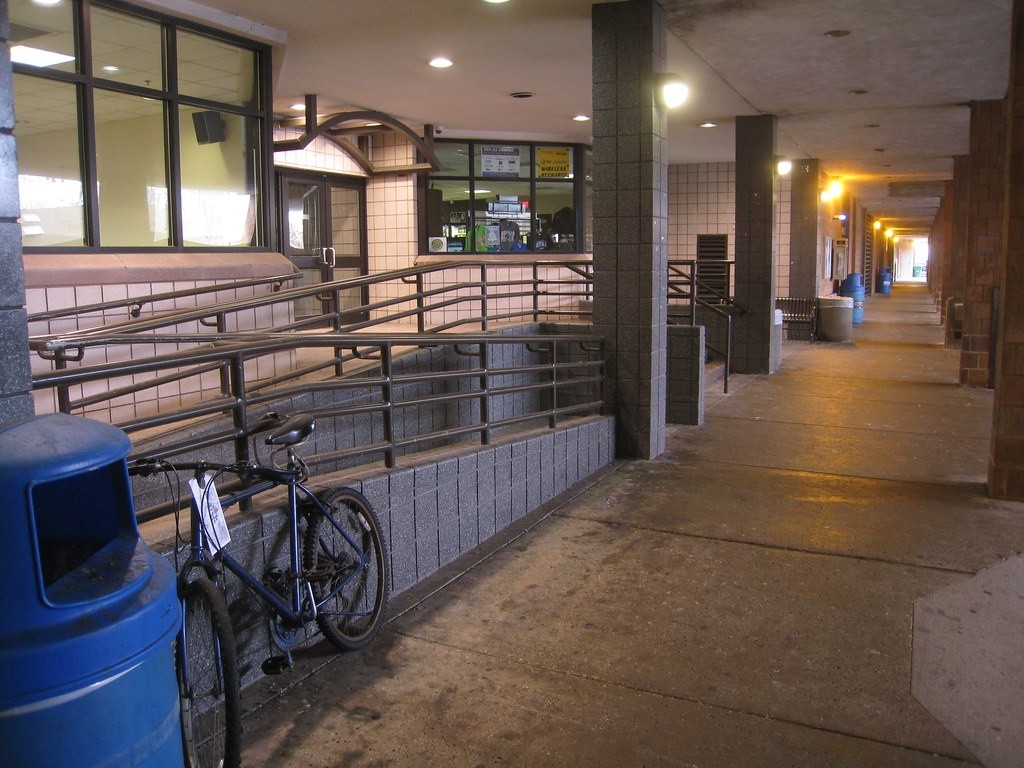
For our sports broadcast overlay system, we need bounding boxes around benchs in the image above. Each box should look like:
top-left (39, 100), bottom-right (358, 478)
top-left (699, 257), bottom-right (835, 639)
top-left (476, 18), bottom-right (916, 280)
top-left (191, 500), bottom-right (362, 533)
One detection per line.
top-left (773, 297), bottom-right (819, 343)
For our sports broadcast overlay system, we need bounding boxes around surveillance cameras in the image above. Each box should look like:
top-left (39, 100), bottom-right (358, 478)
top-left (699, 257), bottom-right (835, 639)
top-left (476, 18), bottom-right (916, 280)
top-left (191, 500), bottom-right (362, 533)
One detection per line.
top-left (435, 126), bottom-right (443, 134)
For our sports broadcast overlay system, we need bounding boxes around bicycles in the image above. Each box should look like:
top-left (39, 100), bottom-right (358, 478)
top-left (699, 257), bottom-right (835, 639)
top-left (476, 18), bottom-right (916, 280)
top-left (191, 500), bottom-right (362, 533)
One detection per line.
top-left (123, 413), bottom-right (391, 768)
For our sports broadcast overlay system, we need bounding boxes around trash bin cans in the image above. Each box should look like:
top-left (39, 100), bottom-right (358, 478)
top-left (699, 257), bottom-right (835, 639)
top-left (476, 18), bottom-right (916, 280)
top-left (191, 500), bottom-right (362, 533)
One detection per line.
top-left (879, 267), bottom-right (892, 294)
top-left (913, 265), bottom-right (922, 277)
top-left (838, 273), bottom-right (865, 324)
top-left (0, 413), bottom-right (185, 768)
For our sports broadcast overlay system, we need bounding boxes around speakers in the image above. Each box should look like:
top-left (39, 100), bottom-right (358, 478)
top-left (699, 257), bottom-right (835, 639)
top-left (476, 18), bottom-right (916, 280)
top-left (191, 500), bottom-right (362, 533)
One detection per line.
top-left (192, 111), bottom-right (226, 144)
